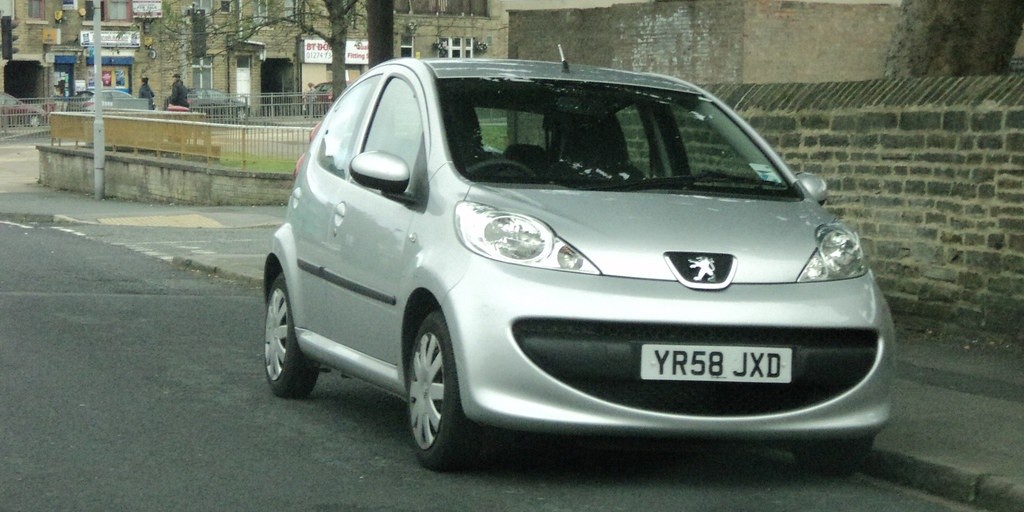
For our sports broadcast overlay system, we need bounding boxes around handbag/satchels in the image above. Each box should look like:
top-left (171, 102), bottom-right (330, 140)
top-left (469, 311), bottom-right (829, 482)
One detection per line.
top-left (150, 89), bottom-right (154, 98)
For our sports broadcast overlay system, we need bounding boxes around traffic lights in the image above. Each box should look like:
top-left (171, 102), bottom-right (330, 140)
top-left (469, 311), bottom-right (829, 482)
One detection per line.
top-left (1, 16), bottom-right (19, 60)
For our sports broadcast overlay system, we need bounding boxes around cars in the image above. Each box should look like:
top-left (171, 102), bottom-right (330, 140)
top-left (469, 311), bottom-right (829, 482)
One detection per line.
top-left (0, 92), bottom-right (47, 127)
top-left (314, 82), bottom-right (351, 113)
top-left (264, 57), bottom-right (897, 480)
top-left (163, 88), bottom-right (251, 120)
top-left (67, 89), bottom-right (136, 112)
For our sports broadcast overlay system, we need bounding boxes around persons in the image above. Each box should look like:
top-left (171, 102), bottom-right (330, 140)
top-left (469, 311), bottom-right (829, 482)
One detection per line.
top-left (139, 77), bottom-right (154, 110)
top-left (304, 82), bottom-right (317, 113)
top-left (163, 74), bottom-right (189, 110)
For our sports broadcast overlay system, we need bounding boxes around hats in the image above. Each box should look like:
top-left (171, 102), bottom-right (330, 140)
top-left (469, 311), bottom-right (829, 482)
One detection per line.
top-left (172, 73), bottom-right (180, 77)
